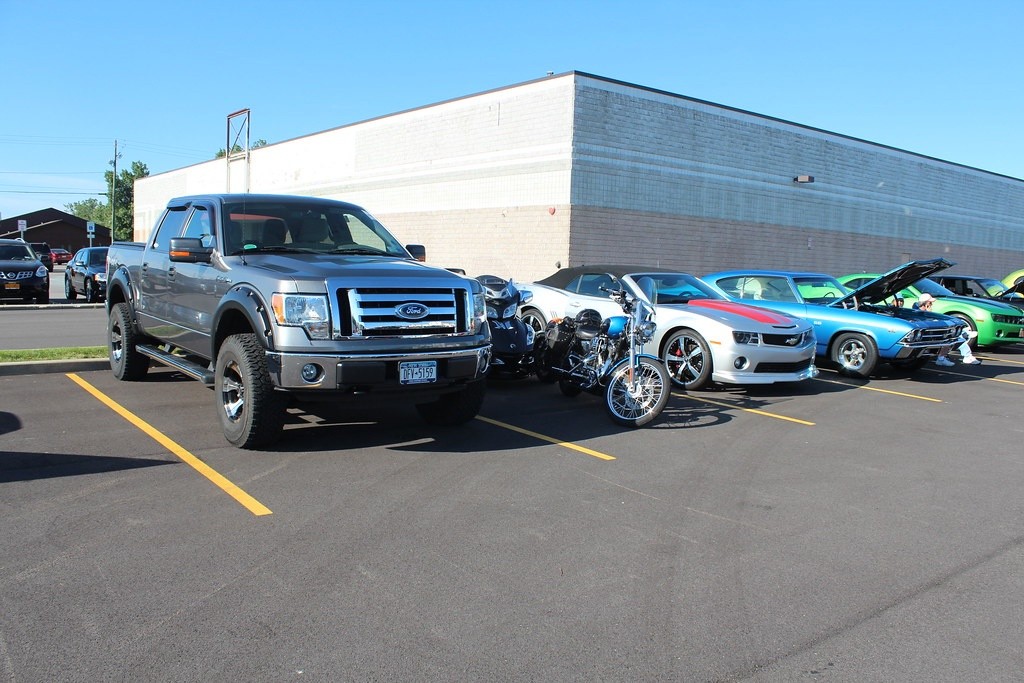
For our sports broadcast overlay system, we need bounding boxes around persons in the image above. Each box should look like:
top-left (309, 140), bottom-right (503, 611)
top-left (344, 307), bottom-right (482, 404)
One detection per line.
top-left (887, 292), bottom-right (955, 367)
top-left (916, 293), bottom-right (981, 365)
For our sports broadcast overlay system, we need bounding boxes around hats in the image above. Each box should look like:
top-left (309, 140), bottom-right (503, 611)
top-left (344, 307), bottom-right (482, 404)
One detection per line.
top-left (892, 292), bottom-right (903, 301)
top-left (918, 293), bottom-right (936, 305)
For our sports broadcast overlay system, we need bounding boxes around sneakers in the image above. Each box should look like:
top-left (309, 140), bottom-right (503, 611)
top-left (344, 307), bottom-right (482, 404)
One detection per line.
top-left (963, 354), bottom-right (981, 365)
top-left (936, 357), bottom-right (955, 367)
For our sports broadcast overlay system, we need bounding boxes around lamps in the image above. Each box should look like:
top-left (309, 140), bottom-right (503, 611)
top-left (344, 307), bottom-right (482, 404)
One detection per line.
top-left (792, 175), bottom-right (815, 184)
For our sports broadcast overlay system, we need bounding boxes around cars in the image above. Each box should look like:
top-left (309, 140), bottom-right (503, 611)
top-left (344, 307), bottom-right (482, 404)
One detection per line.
top-left (795, 273), bottom-right (1024, 350)
top-left (50, 248), bottom-right (73, 265)
top-left (66, 247), bottom-right (108, 302)
top-left (654, 257), bottom-right (968, 381)
top-left (982, 268), bottom-right (1024, 308)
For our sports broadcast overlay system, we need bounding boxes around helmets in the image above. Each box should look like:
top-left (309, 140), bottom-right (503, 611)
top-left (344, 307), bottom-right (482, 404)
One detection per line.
top-left (575, 309), bottom-right (602, 328)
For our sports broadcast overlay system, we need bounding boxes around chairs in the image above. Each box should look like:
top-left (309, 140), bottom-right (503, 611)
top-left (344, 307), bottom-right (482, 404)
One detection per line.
top-left (259, 217), bottom-right (334, 250)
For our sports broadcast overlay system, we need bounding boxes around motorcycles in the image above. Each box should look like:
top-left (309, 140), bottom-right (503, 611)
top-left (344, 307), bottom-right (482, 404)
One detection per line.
top-left (452, 267), bottom-right (671, 428)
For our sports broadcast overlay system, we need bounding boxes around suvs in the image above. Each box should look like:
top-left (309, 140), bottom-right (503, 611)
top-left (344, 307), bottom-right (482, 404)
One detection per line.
top-left (28, 242), bottom-right (53, 273)
top-left (0, 238), bottom-right (50, 304)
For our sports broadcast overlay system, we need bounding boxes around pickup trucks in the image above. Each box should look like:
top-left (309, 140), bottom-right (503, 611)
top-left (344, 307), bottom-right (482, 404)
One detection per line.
top-left (104, 191), bottom-right (494, 450)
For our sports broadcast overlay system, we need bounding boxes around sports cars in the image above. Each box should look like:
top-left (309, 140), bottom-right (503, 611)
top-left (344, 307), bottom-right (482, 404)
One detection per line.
top-left (512, 265), bottom-right (820, 390)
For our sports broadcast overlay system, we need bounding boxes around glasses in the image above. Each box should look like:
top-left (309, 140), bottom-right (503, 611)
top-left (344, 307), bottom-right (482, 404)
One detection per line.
top-left (898, 299), bottom-right (903, 302)
